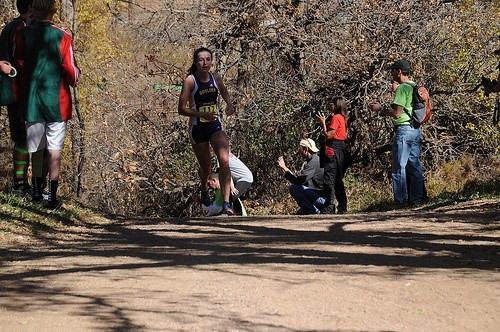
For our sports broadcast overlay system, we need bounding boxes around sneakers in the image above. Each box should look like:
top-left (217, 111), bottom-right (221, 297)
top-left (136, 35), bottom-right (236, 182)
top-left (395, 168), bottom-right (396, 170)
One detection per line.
top-left (201, 191), bottom-right (211, 207)
top-left (218, 202), bottom-right (233, 215)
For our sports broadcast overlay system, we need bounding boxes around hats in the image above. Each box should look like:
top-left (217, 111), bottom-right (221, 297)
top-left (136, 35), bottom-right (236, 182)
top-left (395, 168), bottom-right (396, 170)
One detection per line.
top-left (388, 60), bottom-right (411, 72)
top-left (300, 138), bottom-right (319, 153)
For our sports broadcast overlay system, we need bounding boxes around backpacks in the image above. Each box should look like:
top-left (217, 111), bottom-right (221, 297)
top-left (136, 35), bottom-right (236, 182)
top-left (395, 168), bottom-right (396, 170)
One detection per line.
top-left (401, 82), bottom-right (431, 123)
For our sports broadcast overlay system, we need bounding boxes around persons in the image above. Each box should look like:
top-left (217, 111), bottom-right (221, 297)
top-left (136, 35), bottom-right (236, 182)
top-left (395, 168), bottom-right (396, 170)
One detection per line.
top-left (276, 136), bottom-right (335, 214)
top-left (177, 48), bottom-right (253, 218)
top-left (311, 95), bottom-right (352, 213)
top-left (0, 0), bottom-right (79, 207)
top-left (367, 58), bottom-right (432, 208)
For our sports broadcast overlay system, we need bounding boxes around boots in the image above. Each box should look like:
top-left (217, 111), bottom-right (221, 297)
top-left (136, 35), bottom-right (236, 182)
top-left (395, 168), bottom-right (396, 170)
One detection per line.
top-left (32, 177), bottom-right (62, 209)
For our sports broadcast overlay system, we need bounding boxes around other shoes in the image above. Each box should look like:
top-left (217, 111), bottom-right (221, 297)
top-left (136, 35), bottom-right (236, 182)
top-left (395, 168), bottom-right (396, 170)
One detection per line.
top-left (14, 177), bottom-right (34, 195)
